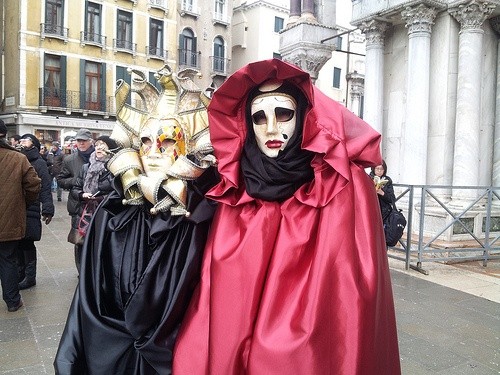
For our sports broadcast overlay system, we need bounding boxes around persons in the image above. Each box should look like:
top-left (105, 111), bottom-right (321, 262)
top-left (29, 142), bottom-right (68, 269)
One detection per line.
top-left (0, 121), bottom-right (394, 311)
top-left (52, 71), bottom-right (220, 375)
top-left (173, 58), bottom-right (402, 375)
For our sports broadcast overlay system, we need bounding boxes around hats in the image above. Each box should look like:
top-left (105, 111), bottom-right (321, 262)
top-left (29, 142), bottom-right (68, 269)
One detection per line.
top-left (75, 129), bottom-right (92, 140)
top-left (96, 135), bottom-right (118, 149)
top-left (53, 142), bottom-right (59, 147)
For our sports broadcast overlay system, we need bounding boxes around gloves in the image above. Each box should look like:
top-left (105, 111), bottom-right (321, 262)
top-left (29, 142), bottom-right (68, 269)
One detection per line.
top-left (73, 177), bottom-right (84, 187)
top-left (42, 214), bottom-right (52, 224)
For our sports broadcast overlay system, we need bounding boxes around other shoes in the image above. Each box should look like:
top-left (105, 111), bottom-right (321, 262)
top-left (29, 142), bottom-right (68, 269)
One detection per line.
top-left (58, 198), bottom-right (61, 201)
top-left (8, 299), bottom-right (24, 312)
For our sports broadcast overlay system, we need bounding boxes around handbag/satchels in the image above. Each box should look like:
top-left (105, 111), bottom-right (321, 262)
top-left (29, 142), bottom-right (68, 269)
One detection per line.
top-left (67, 197), bottom-right (96, 245)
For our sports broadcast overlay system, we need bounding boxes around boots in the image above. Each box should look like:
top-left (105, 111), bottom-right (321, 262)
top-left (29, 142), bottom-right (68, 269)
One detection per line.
top-left (16, 248), bottom-right (37, 289)
